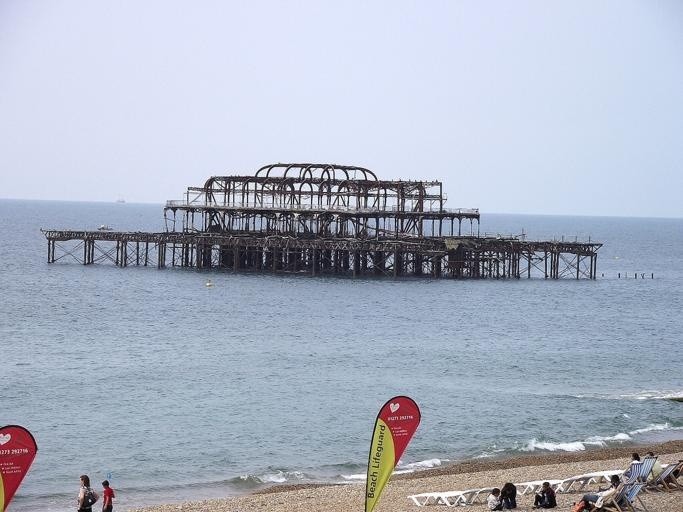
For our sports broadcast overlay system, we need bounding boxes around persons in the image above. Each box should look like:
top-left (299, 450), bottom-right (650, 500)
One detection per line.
top-left (644, 451), bottom-right (654, 457)
top-left (77, 474), bottom-right (100, 512)
top-left (621, 452), bottom-right (641, 484)
top-left (572, 473), bottom-right (620, 512)
top-left (101, 481), bottom-right (115, 512)
top-left (487, 488), bottom-right (502, 510)
top-left (533, 482), bottom-right (555, 509)
top-left (499, 483), bottom-right (518, 510)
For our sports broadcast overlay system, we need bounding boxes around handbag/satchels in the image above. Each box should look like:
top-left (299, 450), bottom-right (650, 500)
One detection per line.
top-left (83, 491), bottom-right (96, 508)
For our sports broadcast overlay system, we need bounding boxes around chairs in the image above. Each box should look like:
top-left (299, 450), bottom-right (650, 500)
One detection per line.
top-left (407, 457), bottom-right (683, 512)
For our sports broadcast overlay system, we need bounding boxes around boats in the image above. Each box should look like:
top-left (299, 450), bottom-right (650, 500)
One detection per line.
top-left (205, 282), bottom-right (213, 287)
top-left (96, 223), bottom-right (115, 231)
